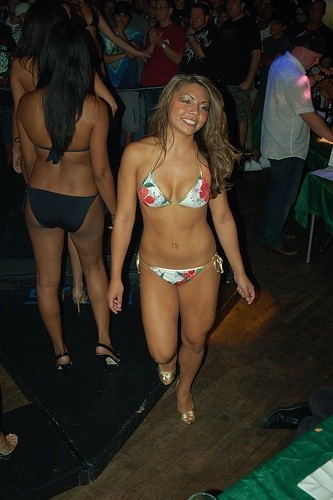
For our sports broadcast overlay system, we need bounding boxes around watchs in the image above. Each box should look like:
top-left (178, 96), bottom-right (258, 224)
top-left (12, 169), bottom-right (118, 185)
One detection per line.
top-left (161, 42), bottom-right (168, 50)
top-left (187, 34), bottom-right (193, 41)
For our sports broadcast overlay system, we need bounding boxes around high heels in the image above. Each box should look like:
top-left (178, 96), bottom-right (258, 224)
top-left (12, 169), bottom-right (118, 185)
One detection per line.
top-left (72, 286), bottom-right (88, 312)
top-left (175, 378), bottom-right (196, 425)
top-left (158, 364), bottom-right (176, 386)
top-left (55, 352), bottom-right (73, 370)
top-left (96, 343), bottom-right (121, 372)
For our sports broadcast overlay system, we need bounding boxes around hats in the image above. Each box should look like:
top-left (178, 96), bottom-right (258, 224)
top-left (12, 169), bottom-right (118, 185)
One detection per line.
top-left (295, 36), bottom-right (327, 55)
top-left (113, 3), bottom-right (131, 16)
top-left (14, 2), bottom-right (31, 17)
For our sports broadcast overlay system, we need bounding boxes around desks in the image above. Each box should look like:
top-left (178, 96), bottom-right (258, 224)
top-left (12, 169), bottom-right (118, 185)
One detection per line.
top-left (216, 413), bottom-right (333, 500)
top-left (206, 160), bottom-right (272, 256)
top-left (294, 165), bottom-right (333, 264)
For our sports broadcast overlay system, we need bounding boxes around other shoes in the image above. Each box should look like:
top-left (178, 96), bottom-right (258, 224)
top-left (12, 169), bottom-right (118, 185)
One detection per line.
top-left (262, 231), bottom-right (297, 241)
top-left (262, 401), bottom-right (313, 429)
top-left (262, 242), bottom-right (302, 256)
top-left (236, 156), bottom-right (271, 171)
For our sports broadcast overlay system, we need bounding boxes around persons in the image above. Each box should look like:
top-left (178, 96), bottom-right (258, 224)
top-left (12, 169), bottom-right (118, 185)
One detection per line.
top-left (105, 76), bottom-right (256, 426)
top-left (0, 0), bottom-right (333, 311)
top-left (0, 429), bottom-right (18, 461)
top-left (258, 383), bottom-right (333, 450)
top-left (17, 21), bottom-right (121, 376)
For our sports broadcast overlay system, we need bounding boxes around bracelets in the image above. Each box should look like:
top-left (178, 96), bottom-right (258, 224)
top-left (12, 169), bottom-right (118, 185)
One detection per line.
top-left (123, 52), bottom-right (127, 57)
